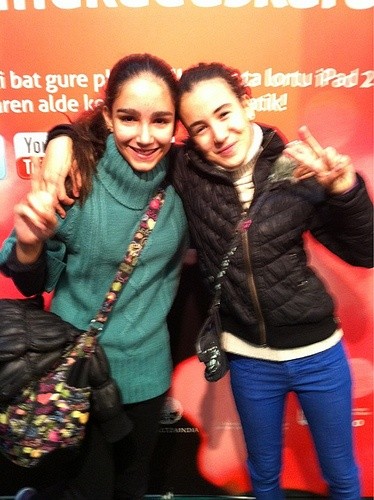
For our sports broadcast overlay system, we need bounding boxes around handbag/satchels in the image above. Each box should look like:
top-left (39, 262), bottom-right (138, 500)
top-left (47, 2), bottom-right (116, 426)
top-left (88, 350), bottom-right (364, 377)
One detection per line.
top-left (0, 337), bottom-right (100, 470)
top-left (192, 308), bottom-right (231, 382)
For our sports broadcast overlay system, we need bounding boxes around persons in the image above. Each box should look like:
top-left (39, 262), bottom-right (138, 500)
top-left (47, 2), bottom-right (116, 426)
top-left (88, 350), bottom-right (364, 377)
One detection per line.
top-left (0, 53), bottom-right (318, 500)
top-left (39, 62), bottom-right (374, 500)
